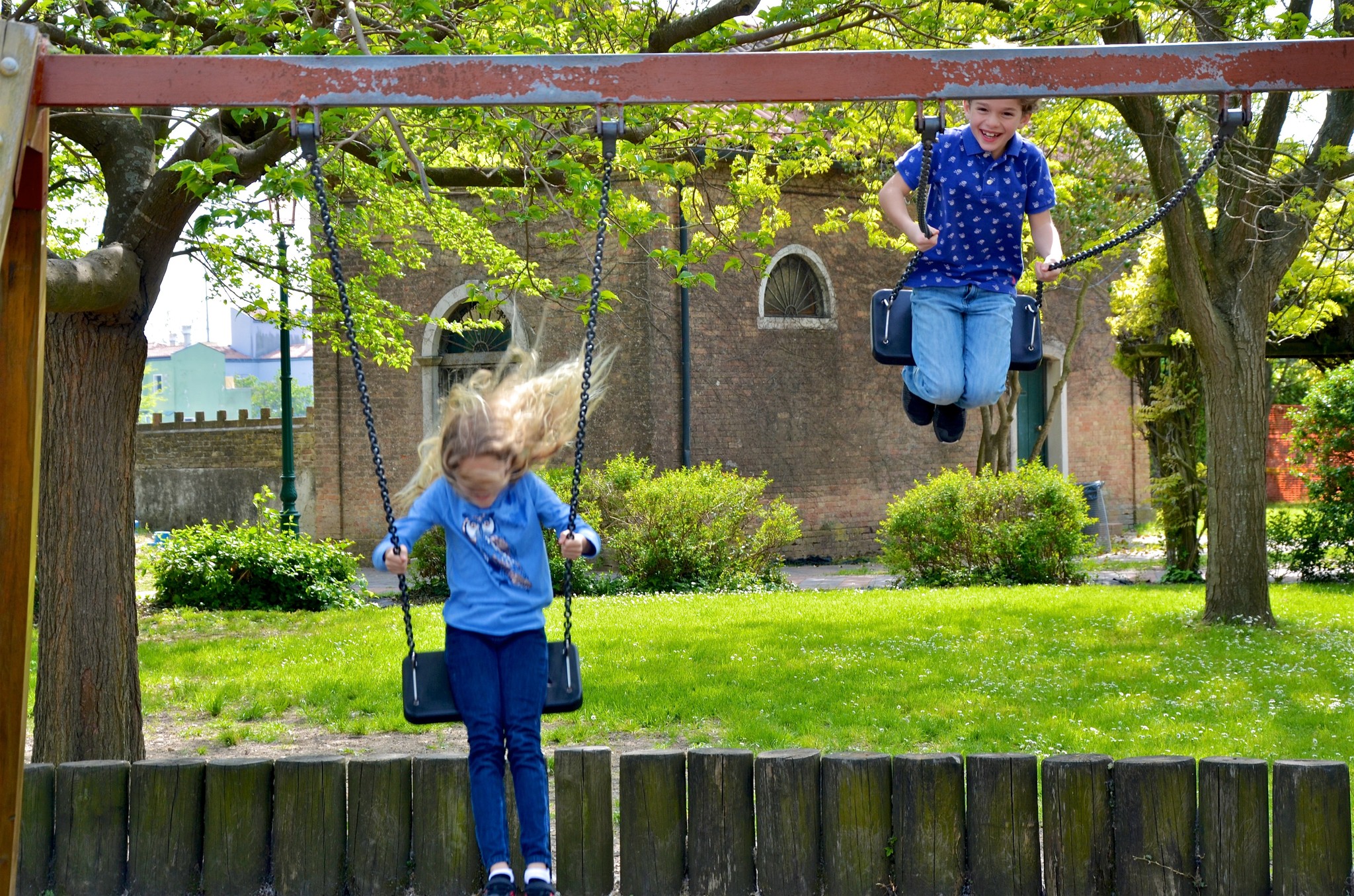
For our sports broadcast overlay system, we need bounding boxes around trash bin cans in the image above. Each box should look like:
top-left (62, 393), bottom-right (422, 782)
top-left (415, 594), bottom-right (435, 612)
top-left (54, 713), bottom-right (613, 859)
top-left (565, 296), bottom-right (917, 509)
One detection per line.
top-left (1077, 479), bottom-right (1111, 556)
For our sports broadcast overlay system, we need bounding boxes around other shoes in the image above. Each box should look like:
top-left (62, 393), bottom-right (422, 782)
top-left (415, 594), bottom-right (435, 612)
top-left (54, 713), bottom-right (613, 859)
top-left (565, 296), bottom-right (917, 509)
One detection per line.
top-left (483, 868), bottom-right (519, 896)
top-left (524, 867), bottom-right (556, 896)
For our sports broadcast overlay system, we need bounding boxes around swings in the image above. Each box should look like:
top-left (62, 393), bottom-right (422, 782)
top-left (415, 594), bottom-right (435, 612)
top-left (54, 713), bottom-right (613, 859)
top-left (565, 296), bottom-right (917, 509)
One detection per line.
top-left (304, 128), bottom-right (610, 725)
top-left (868, 109), bottom-right (1247, 373)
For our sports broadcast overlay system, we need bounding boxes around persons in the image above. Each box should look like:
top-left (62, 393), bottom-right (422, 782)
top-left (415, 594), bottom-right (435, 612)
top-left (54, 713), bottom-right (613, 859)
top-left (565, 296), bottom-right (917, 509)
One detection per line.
top-left (878, 97), bottom-right (1066, 446)
top-left (371, 340), bottom-right (622, 896)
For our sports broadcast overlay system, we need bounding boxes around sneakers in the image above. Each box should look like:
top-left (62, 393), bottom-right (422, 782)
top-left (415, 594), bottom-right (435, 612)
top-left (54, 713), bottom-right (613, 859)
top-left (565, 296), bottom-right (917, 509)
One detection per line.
top-left (933, 402), bottom-right (967, 445)
top-left (901, 381), bottom-right (935, 425)
